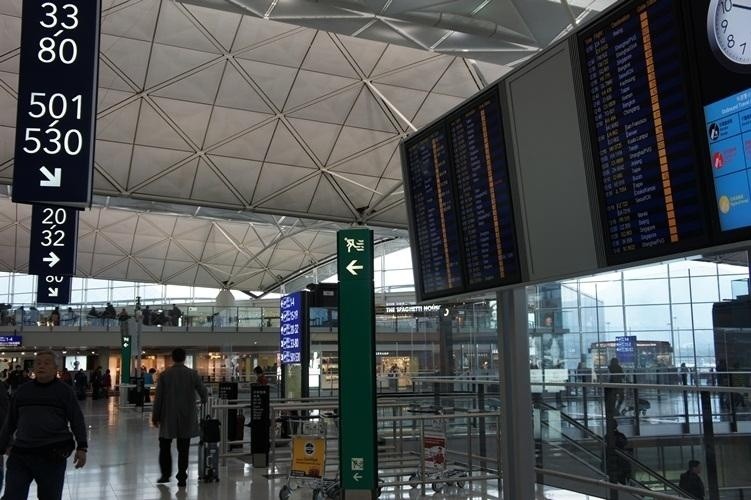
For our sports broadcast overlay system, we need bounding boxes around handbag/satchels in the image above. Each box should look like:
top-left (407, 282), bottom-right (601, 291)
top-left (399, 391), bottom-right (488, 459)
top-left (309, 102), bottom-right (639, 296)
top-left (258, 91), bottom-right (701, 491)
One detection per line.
top-left (200, 414), bottom-right (221, 443)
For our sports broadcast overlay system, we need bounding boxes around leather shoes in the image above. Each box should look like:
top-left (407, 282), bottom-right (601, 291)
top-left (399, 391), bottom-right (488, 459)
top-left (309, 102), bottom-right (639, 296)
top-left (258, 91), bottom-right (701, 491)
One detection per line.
top-left (177, 482), bottom-right (186, 487)
top-left (157, 478), bottom-right (170, 483)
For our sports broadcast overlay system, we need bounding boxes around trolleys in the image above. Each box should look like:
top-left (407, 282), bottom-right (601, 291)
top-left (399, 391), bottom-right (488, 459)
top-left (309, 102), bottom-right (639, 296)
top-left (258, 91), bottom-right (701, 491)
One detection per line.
top-left (275, 415), bottom-right (339, 500)
top-left (407, 410), bottom-right (467, 492)
top-left (621, 376), bottom-right (650, 415)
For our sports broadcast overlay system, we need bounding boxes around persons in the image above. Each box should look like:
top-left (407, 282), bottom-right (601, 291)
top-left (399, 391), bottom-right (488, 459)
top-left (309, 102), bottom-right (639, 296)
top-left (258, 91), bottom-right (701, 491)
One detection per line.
top-left (603, 357), bottom-right (704, 500)
top-left (1, 365), bottom-right (156, 403)
top-left (152, 348), bottom-right (208, 486)
top-left (1, 352), bottom-right (88, 500)
top-left (246, 366), bottom-right (267, 428)
top-left (716, 360), bottom-right (746, 407)
top-left (270, 363), bottom-right (277, 383)
top-left (1, 303), bottom-right (182, 327)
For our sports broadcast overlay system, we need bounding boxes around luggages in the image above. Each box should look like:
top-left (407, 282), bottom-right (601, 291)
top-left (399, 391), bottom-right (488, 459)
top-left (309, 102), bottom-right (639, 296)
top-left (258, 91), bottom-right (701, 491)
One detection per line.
top-left (236, 408), bottom-right (245, 440)
top-left (198, 402), bottom-right (219, 483)
